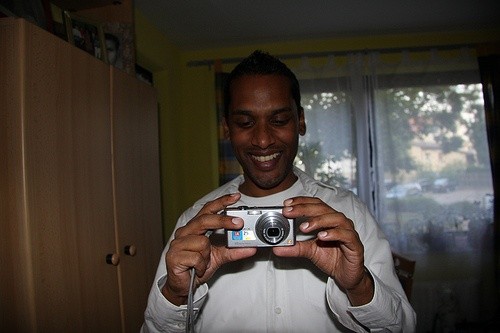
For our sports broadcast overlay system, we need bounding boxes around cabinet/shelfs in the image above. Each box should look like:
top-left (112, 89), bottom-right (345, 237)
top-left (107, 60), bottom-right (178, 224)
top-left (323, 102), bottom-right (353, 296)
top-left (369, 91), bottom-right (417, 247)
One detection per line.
top-left (0, 8), bottom-right (165, 333)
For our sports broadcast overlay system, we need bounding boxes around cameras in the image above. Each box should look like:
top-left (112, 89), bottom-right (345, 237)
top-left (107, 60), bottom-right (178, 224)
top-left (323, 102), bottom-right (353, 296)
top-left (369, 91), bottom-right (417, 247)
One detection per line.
top-left (224, 206), bottom-right (296, 248)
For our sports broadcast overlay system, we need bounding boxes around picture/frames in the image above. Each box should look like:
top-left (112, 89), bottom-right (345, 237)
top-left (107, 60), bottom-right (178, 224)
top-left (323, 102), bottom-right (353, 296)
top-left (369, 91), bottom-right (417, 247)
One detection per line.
top-left (62, 10), bottom-right (109, 65)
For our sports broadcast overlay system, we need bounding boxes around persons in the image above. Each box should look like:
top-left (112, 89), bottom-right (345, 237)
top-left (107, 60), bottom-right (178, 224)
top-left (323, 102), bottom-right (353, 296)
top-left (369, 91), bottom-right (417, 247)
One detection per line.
top-left (139, 50), bottom-right (417, 333)
